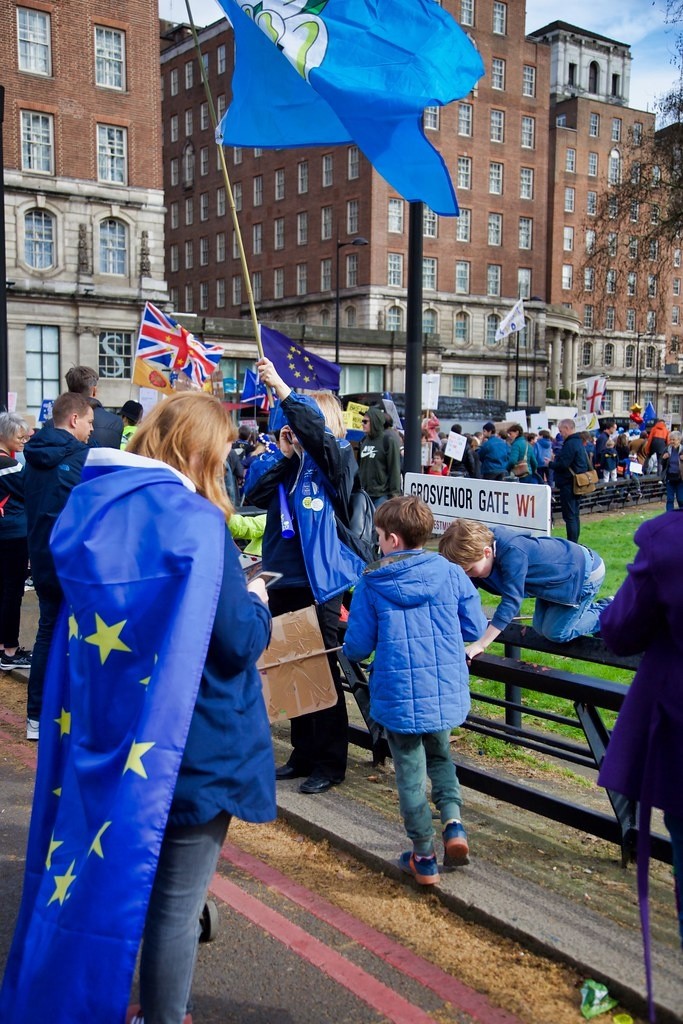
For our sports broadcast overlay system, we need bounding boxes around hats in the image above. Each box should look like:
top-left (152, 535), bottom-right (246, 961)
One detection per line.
top-left (117, 401), bottom-right (143, 423)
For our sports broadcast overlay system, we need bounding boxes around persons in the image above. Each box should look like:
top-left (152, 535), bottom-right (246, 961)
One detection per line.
top-left (0, 366), bottom-right (683, 740)
top-left (245, 362), bottom-right (364, 794)
top-left (601, 507), bottom-right (683, 968)
top-left (341, 494), bottom-right (488, 884)
top-left (0, 390), bottom-right (277, 1023)
top-left (434, 518), bottom-right (616, 661)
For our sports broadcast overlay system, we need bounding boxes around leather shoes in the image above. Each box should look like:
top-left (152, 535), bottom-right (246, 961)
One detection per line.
top-left (274, 763), bottom-right (307, 779)
top-left (300, 774), bottom-right (340, 793)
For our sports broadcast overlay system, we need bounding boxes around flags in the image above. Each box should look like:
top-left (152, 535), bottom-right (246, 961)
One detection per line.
top-left (210, 0), bottom-right (486, 219)
top-left (133, 299), bottom-right (224, 398)
top-left (242, 367), bottom-right (278, 409)
top-left (0, 448), bottom-right (226, 1024)
top-left (639, 401), bottom-right (657, 430)
top-left (494, 299), bottom-right (525, 341)
top-left (582, 376), bottom-right (606, 414)
top-left (258, 325), bottom-right (342, 388)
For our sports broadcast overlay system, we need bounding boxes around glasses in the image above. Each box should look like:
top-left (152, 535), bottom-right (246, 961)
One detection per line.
top-left (361, 419), bottom-right (371, 425)
top-left (8, 435), bottom-right (28, 443)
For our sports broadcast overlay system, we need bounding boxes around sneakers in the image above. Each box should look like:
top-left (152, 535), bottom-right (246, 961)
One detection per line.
top-left (399, 851), bottom-right (440, 884)
top-left (0, 646), bottom-right (35, 669)
top-left (442, 822), bottom-right (471, 867)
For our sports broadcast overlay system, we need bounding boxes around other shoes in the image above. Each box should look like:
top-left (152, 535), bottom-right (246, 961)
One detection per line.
top-left (590, 596), bottom-right (617, 638)
top-left (26, 718), bottom-right (39, 739)
top-left (127, 1003), bottom-right (193, 1024)
top-left (24, 576), bottom-right (35, 592)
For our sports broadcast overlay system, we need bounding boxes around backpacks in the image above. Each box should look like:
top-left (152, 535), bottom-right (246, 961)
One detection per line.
top-left (332, 489), bottom-right (384, 563)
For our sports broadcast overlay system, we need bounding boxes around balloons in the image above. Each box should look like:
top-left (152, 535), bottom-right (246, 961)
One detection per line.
top-left (630, 403), bottom-right (642, 414)
top-left (630, 412), bottom-right (644, 424)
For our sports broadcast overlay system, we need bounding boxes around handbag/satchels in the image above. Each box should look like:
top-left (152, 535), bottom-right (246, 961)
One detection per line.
top-left (512, 461), bottom-right (529, 478)
top-left (573, 470), bottom-right (599, 495)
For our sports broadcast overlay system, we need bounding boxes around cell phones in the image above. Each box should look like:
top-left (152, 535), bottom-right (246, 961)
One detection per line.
top-left (247, 570), bottom-right (284, 588)
top-left (285, 432), bottom-right (293, 444)
